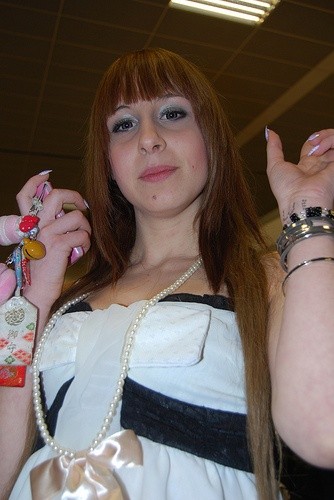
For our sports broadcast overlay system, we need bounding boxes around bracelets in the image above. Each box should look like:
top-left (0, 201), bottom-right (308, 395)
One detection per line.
top-left (274, 205), bottom-right (334, 272)
top-left (281, 257), bottom-right (334, 296)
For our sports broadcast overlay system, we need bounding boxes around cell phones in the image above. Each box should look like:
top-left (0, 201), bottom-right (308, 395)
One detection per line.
top-left (33, 181), bottom-right (86, 270)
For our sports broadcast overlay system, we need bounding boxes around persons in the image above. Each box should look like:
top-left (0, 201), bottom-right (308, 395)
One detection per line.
top-left (0, 45), bottom-right (334, 500)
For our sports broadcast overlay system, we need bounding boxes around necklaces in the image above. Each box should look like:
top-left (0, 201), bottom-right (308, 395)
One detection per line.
top-left (32, 256), bottom-right (203, 460)
